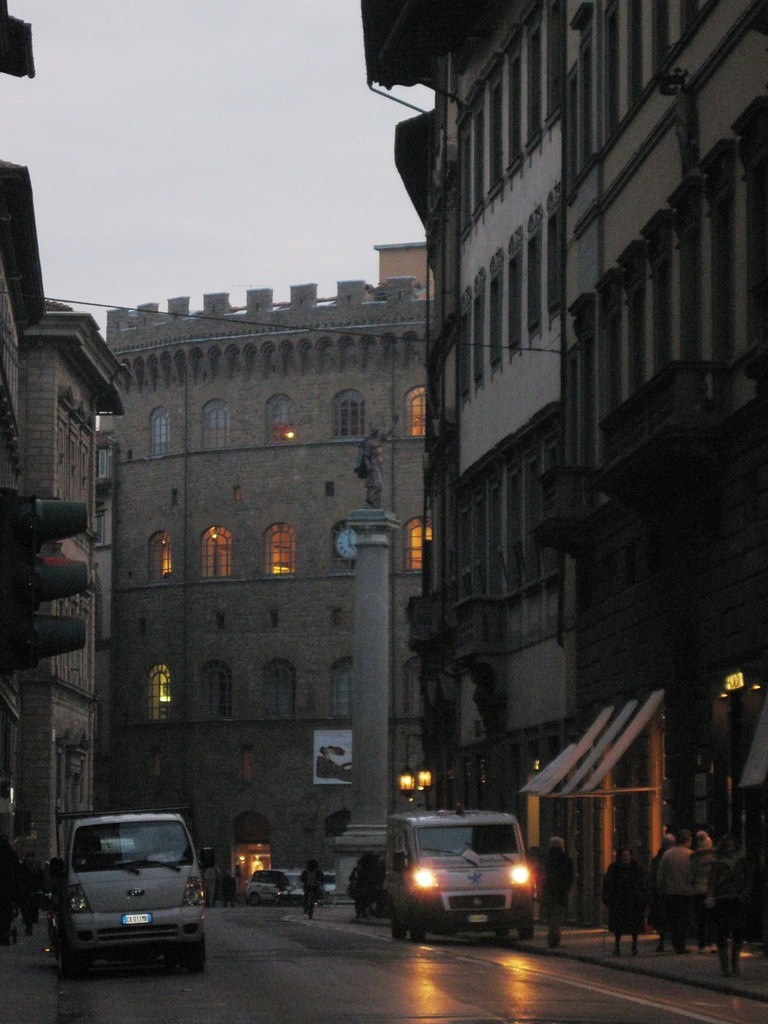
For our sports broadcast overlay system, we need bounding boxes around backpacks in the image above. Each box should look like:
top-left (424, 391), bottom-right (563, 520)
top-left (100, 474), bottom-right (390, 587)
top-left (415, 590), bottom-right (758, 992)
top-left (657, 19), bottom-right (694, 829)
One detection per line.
top-left (305, 868), bottom-right (322, 888)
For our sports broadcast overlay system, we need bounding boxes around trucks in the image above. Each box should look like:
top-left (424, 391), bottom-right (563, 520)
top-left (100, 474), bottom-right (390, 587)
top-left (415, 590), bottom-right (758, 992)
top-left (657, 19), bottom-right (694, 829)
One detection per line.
top-left (46, 810), bottom-right (213, 970)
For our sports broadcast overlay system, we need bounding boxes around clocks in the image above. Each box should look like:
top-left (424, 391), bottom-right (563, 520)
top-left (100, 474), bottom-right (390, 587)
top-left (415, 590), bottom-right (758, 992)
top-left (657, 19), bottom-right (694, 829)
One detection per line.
top-left (335, 527), bottom-right (357, 559)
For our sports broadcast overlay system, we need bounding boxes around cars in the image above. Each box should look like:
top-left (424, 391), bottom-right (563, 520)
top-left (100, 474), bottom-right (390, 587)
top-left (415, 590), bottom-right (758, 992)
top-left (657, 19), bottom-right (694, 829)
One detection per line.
top-left (322, 872), bottom-right (337, 896)
top-left (244, 870), bottom-right (306, 906)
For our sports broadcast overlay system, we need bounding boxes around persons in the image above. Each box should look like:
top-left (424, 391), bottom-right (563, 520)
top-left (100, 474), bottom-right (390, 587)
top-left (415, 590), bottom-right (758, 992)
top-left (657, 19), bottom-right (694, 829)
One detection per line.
top-left (17, 850), bottom-right (44, 934)
top-left (601, 847), bottom-right (649, 957)
top-left (693, 831), bottom-right (718, 953)
top-left (347, 860), bottom-right (370, 920)
top-left (705, 835), bottom-right (753, 976)
top-left (539, 836), bottom-right (577, 948)
top-left (364, 414), bottom-right (399, 509)
top-left (300, 859), bottom-right (325, 920)
top-left (657, 829), bottom-right (697, 954)
top-left (650, 833), bottom-right (676, 952)
top-left (756, 874), bottom-right (768, 958)
top-left (221, 869), bottom-right (237, 908)
top-left (276, 871), bottom-right (289, 892)
top-left (0, 833), bottom-right (20, 945)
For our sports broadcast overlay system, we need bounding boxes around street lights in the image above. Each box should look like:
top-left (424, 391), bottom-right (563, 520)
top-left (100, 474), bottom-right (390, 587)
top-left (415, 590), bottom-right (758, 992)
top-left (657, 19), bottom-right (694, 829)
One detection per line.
top-left (399, 762), bottom-right (433, 811)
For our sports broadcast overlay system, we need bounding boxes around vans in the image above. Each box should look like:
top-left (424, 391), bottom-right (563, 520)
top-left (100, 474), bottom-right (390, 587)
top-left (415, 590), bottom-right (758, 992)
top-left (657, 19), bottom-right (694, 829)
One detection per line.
top-left (384, 802), bottom-right (542, 942)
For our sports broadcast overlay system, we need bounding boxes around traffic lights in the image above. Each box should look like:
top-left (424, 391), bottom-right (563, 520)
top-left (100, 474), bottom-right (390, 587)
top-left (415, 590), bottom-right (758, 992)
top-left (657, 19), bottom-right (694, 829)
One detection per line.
top-left (2, 489), bottom-right (88, 677)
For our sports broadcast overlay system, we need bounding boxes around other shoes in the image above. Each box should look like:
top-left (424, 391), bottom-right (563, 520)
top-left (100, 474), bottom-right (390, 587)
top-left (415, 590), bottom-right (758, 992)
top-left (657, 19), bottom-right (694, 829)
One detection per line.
top-left (723, 969), bottom-right (729, 977)
top-left (305, 914), bottom-right (312, 920)
top-left (656, 949), bottom-right (664, 952)
top-left (675, 949), bottom-right (690, 955)
top-left (730, 958), bottom-right (740, 975)
top-left (613, 951), bottom-right (620, 956)
top-left (632, 948), bottom-right (638, 955)
